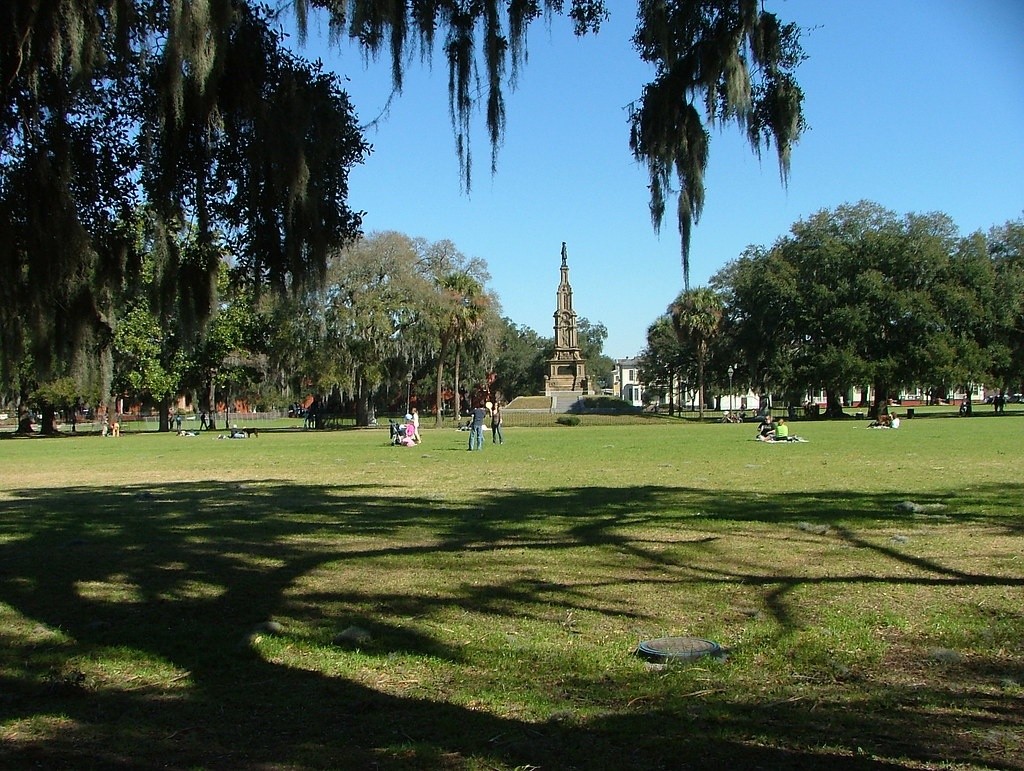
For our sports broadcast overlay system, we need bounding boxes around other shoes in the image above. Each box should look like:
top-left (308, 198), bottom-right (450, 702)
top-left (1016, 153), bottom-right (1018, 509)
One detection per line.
top-left (466, 449), bottom-right (472, 451)
top-left (500, 441), bottom-right (504, 444)
top-left (493, 443), bottom-right (495, 445)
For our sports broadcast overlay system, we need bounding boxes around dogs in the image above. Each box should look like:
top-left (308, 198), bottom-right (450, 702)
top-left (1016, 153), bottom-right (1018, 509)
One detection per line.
top-left (242, 428), bottom-right (262, 438)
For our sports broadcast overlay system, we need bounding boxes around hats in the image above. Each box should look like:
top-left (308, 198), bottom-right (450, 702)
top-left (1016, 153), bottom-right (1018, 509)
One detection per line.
top-left (494, 403), bottom-right (499, 406)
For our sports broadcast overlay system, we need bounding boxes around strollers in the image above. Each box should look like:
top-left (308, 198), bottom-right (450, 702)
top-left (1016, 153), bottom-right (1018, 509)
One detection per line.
top-left (389, 418), bottom-right (408, 447)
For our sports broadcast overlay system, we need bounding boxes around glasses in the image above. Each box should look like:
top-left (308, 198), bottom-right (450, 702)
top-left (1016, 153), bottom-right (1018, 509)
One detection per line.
top-left (765, 418), bottom-right (769, 420)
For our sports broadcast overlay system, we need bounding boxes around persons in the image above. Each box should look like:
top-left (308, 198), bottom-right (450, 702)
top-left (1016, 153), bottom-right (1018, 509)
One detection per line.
top-left (722, 399), bottom-right (900, 442)
top-left (958, 396), bottom-right (1006, 416)
top-left (103, 413), bottom-right (238, 439)
top-left (560, 242), bottom-right (567, 267)
top-left (308, 414), bottom-right (314, 429)
top-left (404, 402), bottom-right (503, 452)
